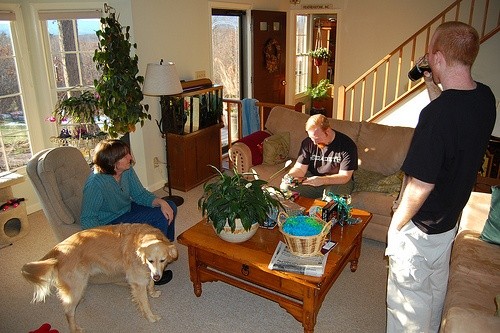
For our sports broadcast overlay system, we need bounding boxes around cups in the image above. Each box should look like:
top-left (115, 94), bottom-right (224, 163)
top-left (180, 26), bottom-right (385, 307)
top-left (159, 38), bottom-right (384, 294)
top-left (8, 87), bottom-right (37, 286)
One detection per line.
top-left (407, 53), bottom-right (432, 82)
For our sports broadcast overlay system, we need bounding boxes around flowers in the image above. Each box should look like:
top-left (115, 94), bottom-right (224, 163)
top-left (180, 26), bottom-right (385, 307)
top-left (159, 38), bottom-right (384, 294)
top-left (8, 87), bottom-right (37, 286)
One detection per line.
top-left (44, 90), bottom-right (102, 132)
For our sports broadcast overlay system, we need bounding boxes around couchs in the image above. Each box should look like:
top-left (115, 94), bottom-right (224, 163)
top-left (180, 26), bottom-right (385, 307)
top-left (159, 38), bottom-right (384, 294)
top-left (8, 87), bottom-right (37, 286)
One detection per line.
top-left (437, 176), bottom-right (500, 333)
top-left (228, 106), bottom-right (415, 243)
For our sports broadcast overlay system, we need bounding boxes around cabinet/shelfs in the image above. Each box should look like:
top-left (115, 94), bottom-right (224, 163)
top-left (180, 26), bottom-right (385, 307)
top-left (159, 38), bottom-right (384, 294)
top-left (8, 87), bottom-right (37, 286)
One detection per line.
top-left (160, 83), bottom-right (225, 192)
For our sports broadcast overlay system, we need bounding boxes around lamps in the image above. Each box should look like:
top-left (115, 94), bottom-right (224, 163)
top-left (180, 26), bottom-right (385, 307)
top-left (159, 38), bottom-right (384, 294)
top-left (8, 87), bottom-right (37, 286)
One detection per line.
top-left (142, 59), bottom-right (184, 207)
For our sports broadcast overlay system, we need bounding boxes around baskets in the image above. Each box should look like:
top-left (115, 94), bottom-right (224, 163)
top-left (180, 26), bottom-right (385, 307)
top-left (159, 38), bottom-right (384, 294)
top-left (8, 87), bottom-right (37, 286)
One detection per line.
top-left (277, 211), bottom-right (335, 258)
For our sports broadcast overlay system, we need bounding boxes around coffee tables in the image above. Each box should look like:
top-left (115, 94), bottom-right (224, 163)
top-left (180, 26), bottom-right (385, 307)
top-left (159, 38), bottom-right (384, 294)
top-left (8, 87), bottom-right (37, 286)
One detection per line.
top-left (177, 187), bottom-right (373, 333)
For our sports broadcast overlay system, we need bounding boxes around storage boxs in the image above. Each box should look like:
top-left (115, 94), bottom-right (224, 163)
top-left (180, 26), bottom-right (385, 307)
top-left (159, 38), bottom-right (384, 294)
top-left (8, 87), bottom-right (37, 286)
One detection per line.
top-left (322, 200), bottom-right (339, 222)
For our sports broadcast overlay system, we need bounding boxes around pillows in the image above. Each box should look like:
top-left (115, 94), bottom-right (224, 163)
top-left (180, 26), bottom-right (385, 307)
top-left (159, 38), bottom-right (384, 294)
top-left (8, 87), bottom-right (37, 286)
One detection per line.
top-left (261, 131), bottom-right (290, 167)
top-left (479, 185), bottom-right (500, 245)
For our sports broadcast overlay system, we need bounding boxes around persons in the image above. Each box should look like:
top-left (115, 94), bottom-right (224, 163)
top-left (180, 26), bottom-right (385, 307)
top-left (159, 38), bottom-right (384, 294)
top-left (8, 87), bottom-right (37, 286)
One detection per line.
top-left (78, 139), bottom-right (178, 285)
top-left (384, 21), bottom-right (497, 333)
top-left (278, 114), bottom-right (358, 207)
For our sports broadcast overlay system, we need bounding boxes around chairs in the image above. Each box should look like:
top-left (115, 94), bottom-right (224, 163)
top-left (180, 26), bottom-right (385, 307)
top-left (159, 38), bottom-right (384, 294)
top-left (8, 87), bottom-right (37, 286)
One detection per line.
top-left (26, 146), bottom-right (93, 243)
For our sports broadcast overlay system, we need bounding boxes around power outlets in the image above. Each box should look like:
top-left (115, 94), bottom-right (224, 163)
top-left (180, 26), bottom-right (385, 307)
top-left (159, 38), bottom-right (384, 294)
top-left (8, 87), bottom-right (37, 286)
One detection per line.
top-left (154, 158), bottom-right (159, 168)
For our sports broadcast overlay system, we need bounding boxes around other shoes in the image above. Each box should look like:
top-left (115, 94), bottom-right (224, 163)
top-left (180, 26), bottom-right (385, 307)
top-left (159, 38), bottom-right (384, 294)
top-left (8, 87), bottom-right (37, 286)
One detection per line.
top-left (154, 269), bottom-right (173, 285)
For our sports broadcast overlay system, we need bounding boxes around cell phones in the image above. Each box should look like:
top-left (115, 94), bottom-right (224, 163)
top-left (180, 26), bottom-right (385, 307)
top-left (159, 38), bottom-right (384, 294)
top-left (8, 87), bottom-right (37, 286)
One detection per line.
top-left (320, 241), bottom-right (338, 256)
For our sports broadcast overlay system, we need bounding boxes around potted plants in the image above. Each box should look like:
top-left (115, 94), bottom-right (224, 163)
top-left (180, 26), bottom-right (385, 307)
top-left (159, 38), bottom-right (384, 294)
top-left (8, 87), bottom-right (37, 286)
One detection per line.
top-left (304, 79), bottom-right (330, 110)
top-left (197, 164), bottom-right (287, 242)
top-left (296, 46), bottom-right (333, 66)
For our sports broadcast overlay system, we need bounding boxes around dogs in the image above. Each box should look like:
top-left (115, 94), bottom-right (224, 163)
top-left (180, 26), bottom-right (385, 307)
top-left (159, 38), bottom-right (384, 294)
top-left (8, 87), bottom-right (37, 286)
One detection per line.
top-left (20, 222), bottom-right (179, 333)
